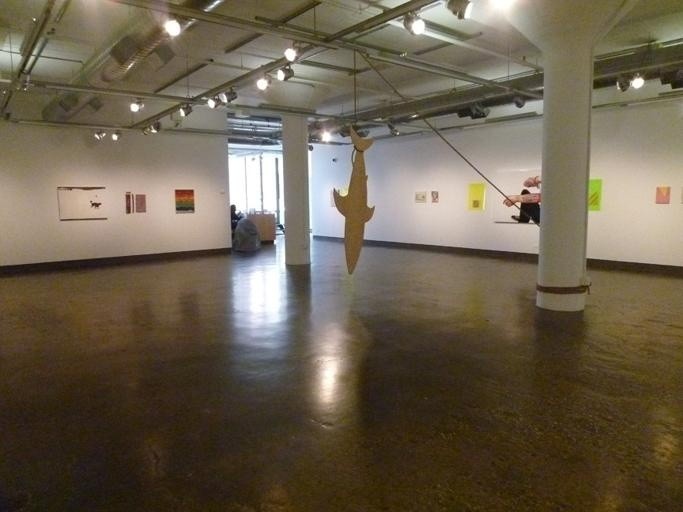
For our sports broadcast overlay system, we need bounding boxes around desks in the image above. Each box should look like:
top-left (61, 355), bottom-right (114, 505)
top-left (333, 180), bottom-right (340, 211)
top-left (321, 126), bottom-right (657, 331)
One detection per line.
top-left (247, 213), bottom-right (276, 246)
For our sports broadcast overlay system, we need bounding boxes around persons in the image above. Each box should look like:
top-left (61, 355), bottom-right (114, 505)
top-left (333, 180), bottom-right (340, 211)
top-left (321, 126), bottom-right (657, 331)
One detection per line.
top-left (230, 203), bottom-right (242, 232)
top-left (502, 173), bottom-right (541, 226)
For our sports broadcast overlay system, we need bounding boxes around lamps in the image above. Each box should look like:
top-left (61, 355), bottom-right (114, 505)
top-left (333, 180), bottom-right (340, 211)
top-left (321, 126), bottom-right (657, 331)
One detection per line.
top-left (175, 39), bottom-right (305, 124)
top-left (404, 1), bottom-right (471, 36)
top-left (92, 97), bottom-right (162, 145)
top-left (616, 72), bottom-right (646, 93)
